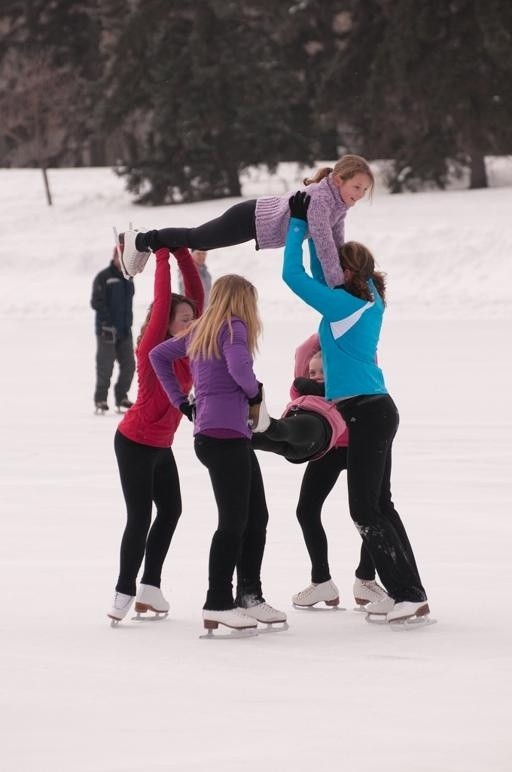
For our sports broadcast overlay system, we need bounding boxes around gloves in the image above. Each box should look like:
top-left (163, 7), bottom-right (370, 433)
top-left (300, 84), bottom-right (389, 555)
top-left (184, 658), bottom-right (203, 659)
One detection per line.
top-left (143, 230), bottom-right (162, 253)
top-left (179, 401), bottom-right (196, 421)
top-left (289, 191), bottom-right (310, 220)
top-left (294, 377), bottom-right (321, 396)
top-left (248, 383), bottom-right (262, 406)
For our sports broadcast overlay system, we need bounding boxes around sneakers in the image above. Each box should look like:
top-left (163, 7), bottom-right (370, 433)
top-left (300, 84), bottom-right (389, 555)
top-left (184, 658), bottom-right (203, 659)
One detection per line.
top-left (119, 229), bottom-right (150, 281)
top-left (248, 391), bottom-right (271, 433)
top-left (108, 583), bottom-right (169, 620)
top-left (202, 579), bottom-right (429, 629)
top-left (97, 396), bottom-right (133, 410)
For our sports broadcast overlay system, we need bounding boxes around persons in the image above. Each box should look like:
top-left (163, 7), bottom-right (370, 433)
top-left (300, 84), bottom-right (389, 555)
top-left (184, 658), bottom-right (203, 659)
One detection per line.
top-left (289, 396), bottom-right (395, 609)
top-left (88, 243), bottom-right (137, 411)
top-left (104, 246), bottom-right (207, 622)
top-left (148, 274), bottom-right (291, 629)
top-left (175, 244), bottom-right (211, 306)
top-left (281, 190), bottom-right (431, 624)
top-left (121, 152), bottom-right (375, 289)
top-left (231, 332), bottom-right (350, 464)
